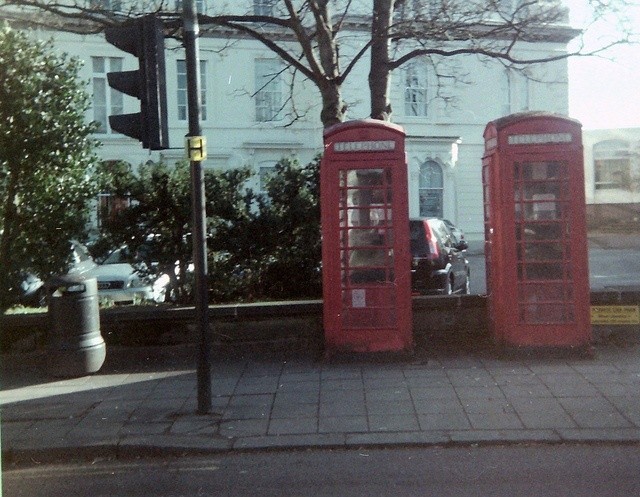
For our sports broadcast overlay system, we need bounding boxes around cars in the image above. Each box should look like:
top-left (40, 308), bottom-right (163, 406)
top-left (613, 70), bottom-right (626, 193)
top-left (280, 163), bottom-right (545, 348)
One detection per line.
top-left (96, 231), bottom-right (195, 304)
top-left (14, 239), bottom-right (92, 307)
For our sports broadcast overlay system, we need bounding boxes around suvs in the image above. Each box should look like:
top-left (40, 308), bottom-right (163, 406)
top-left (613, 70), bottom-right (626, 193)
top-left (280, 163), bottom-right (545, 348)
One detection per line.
top-left (351, 215), bottom-right (473, 296)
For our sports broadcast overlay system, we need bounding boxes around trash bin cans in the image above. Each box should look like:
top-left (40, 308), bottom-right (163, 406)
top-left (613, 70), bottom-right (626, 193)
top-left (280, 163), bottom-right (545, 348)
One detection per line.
top-left (47, 276), bottom-right (107, 377)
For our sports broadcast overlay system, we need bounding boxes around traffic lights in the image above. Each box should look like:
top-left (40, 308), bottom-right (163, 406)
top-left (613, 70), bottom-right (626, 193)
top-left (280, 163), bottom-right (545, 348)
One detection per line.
top-left (105, 16), bottom-right (171, 150)
